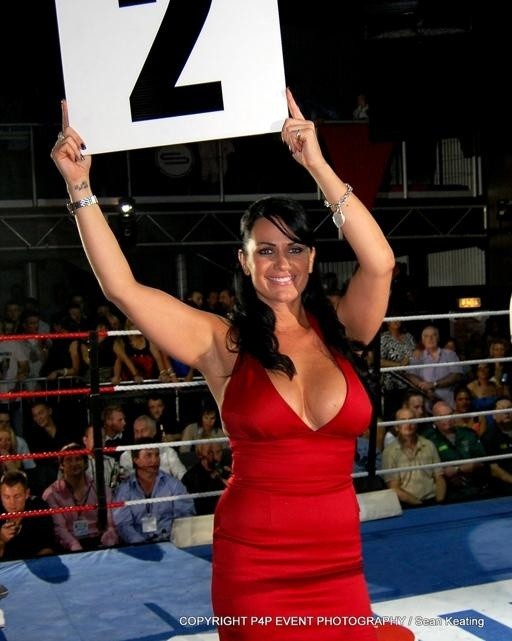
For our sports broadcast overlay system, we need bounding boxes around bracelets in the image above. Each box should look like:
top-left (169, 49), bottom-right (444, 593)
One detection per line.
top-left (66, 195), bottom-right (99, 216)
top-left (324, 182), bottom-right (354, 243)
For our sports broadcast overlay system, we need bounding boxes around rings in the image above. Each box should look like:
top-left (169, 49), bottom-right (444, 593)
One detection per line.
top-left (297, 129), bottom-right (300, 138)
top-left (57, 131), bottom-right (65, 140)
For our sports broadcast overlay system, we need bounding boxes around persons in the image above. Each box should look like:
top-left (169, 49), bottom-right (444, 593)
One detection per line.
top-left (353, 94), bottom-right (369, 121)
top-left (49, 88), bottom-right (397, 639)
